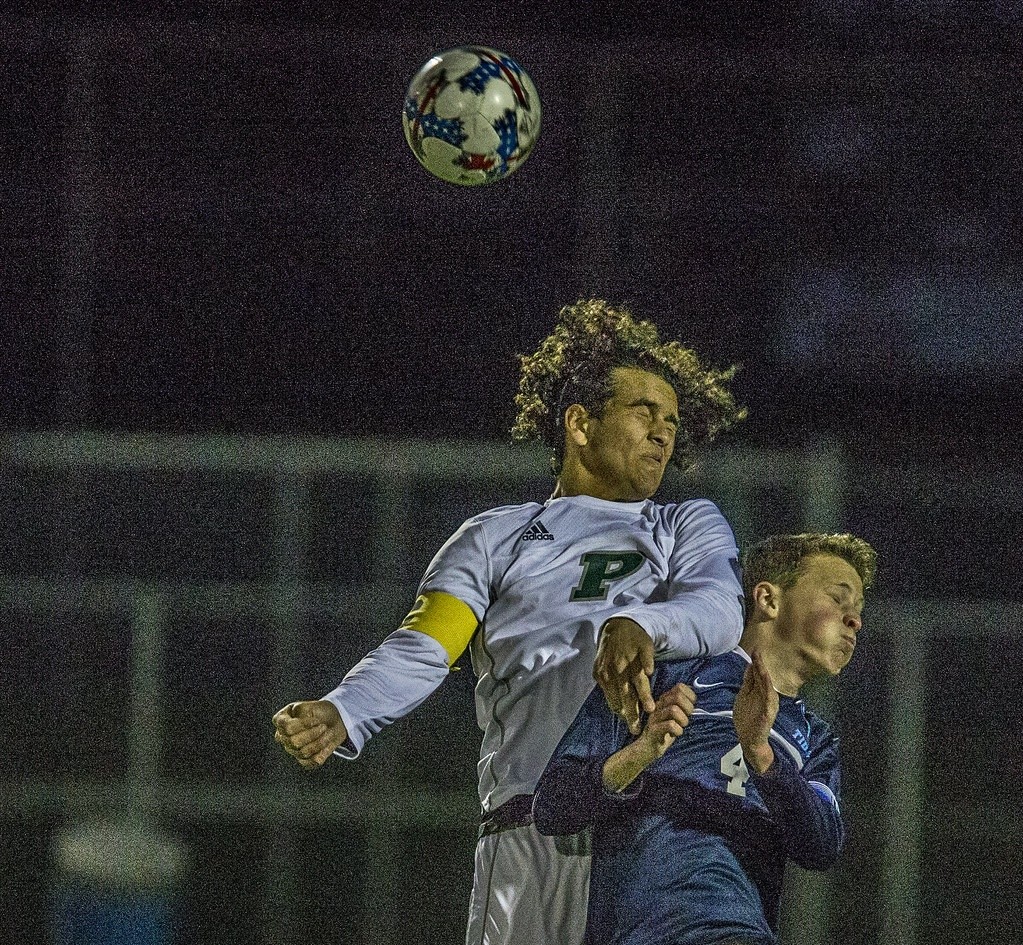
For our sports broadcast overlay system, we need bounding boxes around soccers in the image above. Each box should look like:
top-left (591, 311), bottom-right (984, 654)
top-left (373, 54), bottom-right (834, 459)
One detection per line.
top-left (401, 45), bottom-right (542, 187)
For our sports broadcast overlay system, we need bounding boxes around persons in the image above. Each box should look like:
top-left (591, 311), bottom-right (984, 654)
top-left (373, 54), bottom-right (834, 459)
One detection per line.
top-left (532, 535), bottom-right (878, 945)
top-left (272, 302), bottom-right (746, 945)
